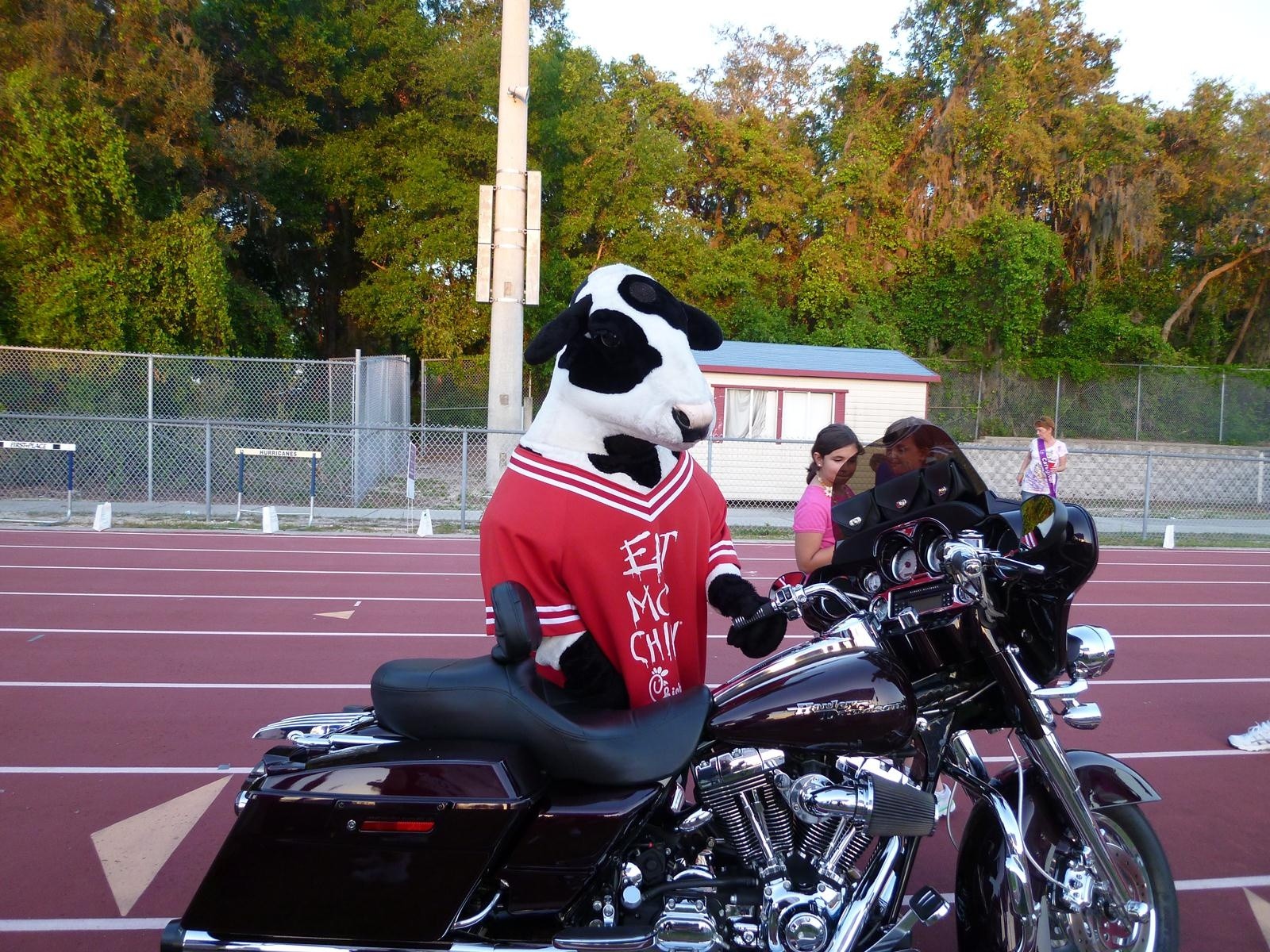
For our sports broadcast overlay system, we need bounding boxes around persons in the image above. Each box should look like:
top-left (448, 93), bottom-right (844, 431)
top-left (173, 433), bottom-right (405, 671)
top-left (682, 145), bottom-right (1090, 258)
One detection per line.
top-left (884, 416), bottom-right (956, 819)
top-left (793, 423), bottom-right (863, 583)
top-left (1017, 416), bottom-right (1069, 501)
top-left (1229, 719), bottom-right (1270, 753)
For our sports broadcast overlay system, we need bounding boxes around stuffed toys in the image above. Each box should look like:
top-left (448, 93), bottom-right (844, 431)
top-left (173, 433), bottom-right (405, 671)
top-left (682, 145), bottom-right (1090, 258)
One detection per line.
top-left (479, 264), bottom-right (788, 711)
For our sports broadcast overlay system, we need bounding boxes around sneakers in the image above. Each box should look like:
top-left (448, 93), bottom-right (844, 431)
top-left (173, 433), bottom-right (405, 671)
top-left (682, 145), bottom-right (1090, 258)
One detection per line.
top-left (1226, 719), bottom-right (1270, 751)
top-left (932, 782), bottom-right (958, 821)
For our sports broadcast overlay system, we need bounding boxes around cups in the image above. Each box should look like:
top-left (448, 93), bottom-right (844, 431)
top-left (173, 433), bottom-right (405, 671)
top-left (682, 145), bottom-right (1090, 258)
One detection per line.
top-left (1048, 460), bottom-right (1055, 470)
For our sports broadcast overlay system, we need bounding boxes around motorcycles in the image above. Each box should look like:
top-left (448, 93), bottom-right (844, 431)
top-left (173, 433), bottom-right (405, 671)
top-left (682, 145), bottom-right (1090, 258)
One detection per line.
top-left (162, 423), bottom-right (1182, 952)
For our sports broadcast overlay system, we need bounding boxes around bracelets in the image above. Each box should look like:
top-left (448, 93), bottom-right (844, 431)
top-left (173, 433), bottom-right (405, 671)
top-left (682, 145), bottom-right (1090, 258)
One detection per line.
top-left (1018, 472), bottom-right (1024, 475)
